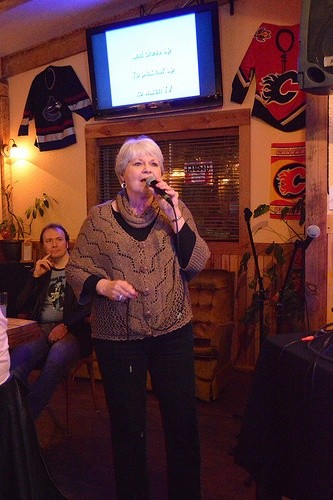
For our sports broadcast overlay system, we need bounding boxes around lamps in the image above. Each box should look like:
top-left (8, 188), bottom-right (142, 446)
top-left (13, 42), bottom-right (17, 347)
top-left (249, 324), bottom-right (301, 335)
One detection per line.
top-left (1, 138), bottom-right (21, 158)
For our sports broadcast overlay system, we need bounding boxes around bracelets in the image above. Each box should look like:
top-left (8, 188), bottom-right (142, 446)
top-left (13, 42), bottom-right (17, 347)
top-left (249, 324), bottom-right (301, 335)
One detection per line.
top-left (169, 215), bottom-right (183, 222)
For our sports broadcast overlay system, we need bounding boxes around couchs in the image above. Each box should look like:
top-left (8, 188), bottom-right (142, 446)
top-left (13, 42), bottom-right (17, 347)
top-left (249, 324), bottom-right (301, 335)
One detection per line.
top-left (76, 267), bottom-right (236, 401)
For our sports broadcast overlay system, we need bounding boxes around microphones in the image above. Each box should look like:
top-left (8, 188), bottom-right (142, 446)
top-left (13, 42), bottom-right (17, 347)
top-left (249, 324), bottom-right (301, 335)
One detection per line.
top-left (302, 225), bottom-right (321, 250)
top-left (145, 176), bottom-right (174, 208)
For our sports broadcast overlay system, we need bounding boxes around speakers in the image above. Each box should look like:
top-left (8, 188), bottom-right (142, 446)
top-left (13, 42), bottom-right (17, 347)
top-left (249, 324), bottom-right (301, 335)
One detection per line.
top-left (299, 0), bottom-right (333, 95)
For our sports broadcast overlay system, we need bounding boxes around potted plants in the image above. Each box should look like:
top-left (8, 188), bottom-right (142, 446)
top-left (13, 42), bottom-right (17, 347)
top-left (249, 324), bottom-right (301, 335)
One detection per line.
top-left (0, 184), bottom-right (59, 263)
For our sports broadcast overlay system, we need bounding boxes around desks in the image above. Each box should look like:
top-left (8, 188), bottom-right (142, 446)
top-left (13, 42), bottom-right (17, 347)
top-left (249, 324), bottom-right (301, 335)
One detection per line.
top-left (232, 332), bottom-right (333, 500)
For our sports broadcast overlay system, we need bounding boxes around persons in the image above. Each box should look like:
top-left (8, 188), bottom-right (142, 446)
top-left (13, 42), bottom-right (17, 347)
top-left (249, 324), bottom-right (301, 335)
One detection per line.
top-left (65, 134), bottom-right (210, 500)
top-left (0, 308), bottom-right (10, 385)
top-left (6, 223), bottom-right (93, 419)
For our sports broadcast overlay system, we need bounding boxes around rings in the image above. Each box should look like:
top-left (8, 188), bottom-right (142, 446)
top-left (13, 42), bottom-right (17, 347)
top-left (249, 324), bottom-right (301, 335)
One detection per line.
top-left (119, 295), bottom-right (122, 300)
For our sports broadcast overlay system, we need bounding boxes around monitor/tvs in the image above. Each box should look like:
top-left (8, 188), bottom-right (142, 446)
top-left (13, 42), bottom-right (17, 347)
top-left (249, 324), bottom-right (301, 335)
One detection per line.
top-left (86, 2), bottom-right (223, 121)
